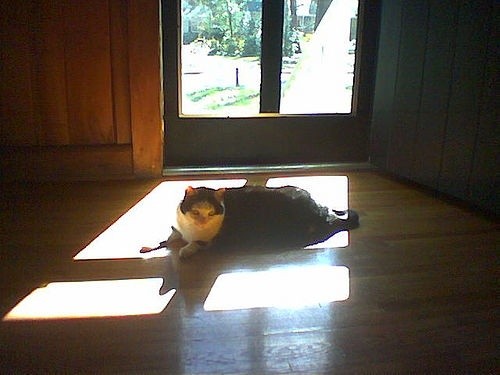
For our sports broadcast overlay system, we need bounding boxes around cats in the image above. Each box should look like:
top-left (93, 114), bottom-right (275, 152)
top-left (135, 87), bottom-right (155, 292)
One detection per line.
top-left (167, 186), bottom-right (358, 259)
